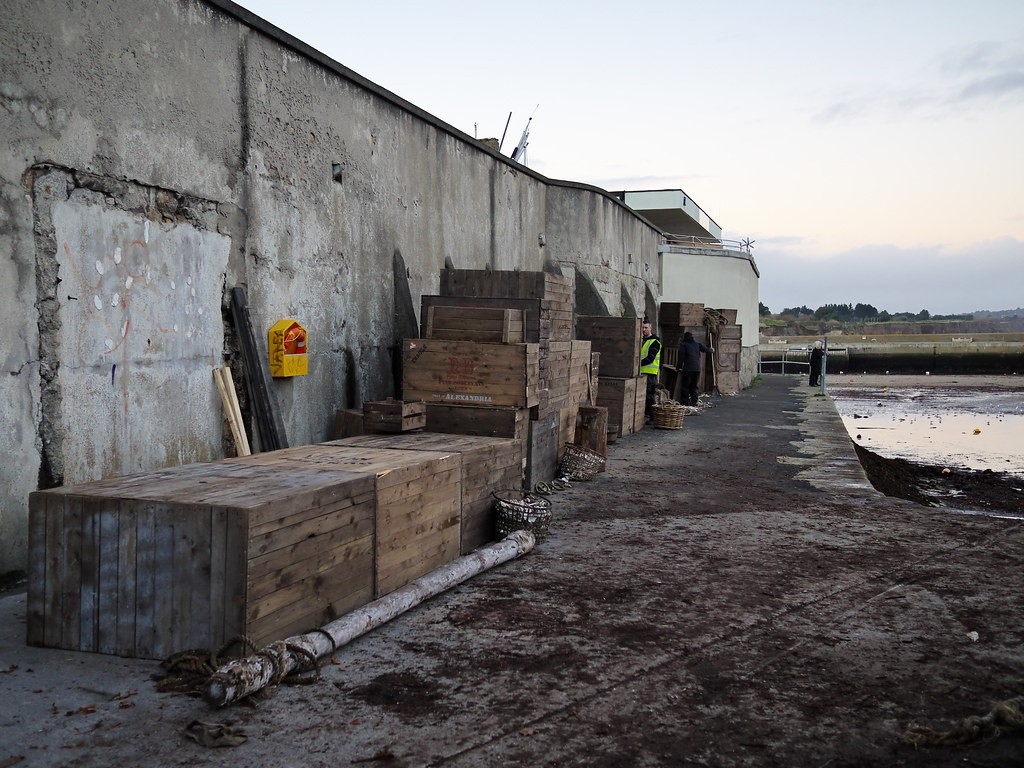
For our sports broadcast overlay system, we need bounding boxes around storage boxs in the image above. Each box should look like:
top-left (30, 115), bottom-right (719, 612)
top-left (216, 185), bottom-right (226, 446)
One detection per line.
top-left (23, 266), bottom-right (742, 660)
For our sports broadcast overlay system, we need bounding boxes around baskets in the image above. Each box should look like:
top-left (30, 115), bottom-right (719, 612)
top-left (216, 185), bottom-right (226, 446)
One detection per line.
top-left (493, 489), bottom-right (553, 544)
top-left (652, 400), bottom-right (686, 429)
top-left (559, 441), bottom-right (607, 481)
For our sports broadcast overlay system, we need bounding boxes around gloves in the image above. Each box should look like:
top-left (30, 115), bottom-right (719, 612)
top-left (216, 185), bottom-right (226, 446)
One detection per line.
top-left (676, 367), bottom-right (680, 370)
top-left (709, 347), bottom-right (714, 353)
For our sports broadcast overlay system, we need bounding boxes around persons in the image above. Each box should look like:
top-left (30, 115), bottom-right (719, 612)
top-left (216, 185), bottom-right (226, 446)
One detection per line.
top-left (675, 331), bottom-right (715, 406)
top-left (641, 320), bottom-right (662, 424)
top-left (809, 341), bottom-right (825, 387)
